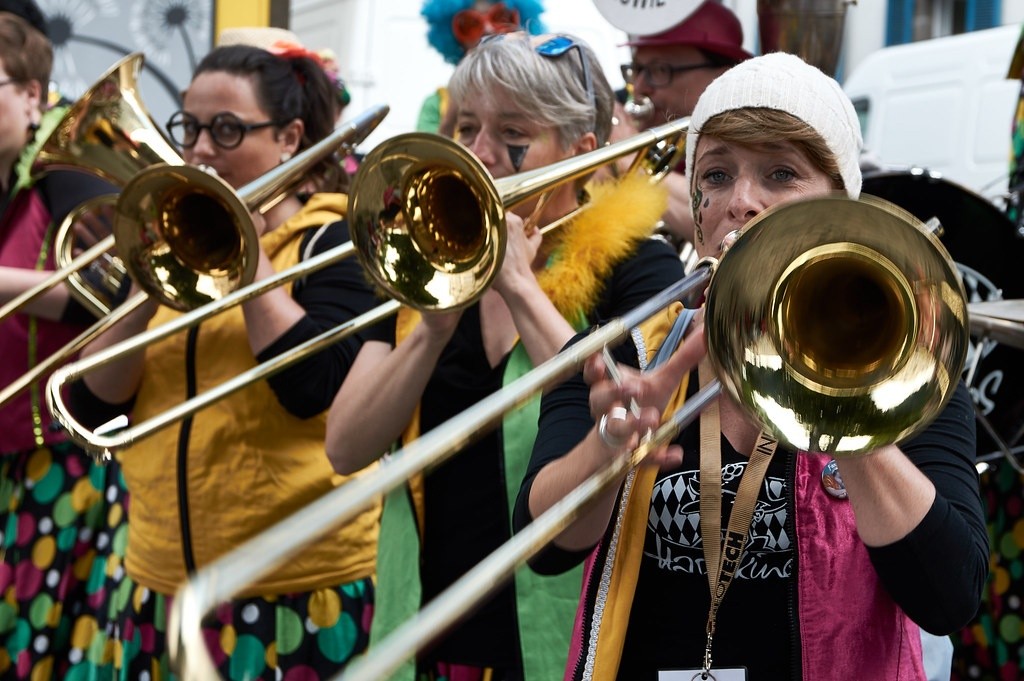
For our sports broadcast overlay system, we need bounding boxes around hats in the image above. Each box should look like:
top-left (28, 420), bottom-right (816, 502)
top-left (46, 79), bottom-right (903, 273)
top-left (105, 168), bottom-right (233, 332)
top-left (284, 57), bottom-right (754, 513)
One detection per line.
top-left (595, 0), bottom-right (755, 55)
top-left (219, 27), bottom-right (321, 63)
top-left (685, 51), bottom-right (865, 220)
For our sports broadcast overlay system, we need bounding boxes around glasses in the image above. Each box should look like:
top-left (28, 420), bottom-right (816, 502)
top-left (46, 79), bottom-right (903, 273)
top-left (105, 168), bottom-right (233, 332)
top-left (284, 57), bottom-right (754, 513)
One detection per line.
top-left (453, 4), bottom-right (520, 46)
top-left (620, 59), bottom-right (736, 86)
top-left (166, 107), bottom-right (282, 149)
top-left (480, 33), bottom-right (596, 134)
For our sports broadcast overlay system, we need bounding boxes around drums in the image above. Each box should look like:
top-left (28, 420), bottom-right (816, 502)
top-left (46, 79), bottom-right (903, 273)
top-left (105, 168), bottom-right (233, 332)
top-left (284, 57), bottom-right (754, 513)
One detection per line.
top-left (852, 164), bottom-right (1024, 472)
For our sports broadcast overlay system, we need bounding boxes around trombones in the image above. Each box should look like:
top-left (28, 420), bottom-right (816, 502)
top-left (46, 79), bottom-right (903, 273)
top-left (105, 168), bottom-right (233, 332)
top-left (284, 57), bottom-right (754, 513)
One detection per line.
top-left (42, 112), bottom-right (693, 460)
top-left (1, 102), bottom-right (393, 409)
top-left (167, 186), bottom-right (972, 681)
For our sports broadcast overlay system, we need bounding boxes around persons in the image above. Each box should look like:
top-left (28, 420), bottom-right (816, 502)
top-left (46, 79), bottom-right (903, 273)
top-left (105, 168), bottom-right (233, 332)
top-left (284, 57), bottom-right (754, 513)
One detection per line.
top-left (1, 0), bottom-right (1024, 681)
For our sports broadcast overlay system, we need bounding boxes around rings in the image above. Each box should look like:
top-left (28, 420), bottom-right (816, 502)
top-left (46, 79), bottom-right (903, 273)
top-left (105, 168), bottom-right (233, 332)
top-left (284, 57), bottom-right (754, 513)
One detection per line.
top-left (599, 405), bottom-right (633, 449)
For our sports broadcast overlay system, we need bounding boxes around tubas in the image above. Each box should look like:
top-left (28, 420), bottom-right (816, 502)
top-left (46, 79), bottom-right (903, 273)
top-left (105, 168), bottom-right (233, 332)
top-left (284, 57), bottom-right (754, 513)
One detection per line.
top-left (28, 47), bottom-right (190, 322)
